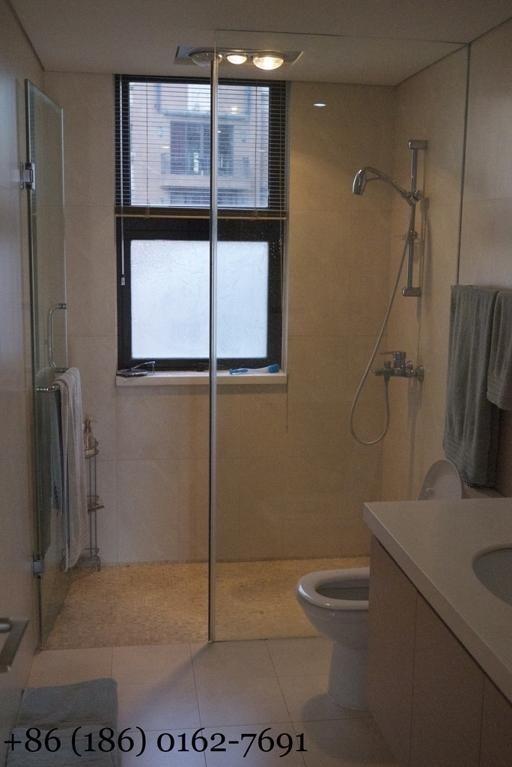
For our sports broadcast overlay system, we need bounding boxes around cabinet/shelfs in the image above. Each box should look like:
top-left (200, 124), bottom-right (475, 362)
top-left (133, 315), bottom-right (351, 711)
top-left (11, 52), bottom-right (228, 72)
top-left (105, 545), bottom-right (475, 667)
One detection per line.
top-left (369, 522), bottom-right (507, 766)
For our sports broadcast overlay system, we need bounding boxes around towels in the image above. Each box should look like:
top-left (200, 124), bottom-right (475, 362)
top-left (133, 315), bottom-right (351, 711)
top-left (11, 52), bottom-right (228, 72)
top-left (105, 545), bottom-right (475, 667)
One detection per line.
top-left (49, 365), bottom-right (92, 571)
top-left (442, 283), bottom-right (511, 489)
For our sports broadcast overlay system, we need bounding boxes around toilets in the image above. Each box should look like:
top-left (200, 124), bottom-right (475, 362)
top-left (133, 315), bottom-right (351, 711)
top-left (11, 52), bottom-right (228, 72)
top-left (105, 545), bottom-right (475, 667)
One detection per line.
top-left (298, 460), bottom-right (463, 711)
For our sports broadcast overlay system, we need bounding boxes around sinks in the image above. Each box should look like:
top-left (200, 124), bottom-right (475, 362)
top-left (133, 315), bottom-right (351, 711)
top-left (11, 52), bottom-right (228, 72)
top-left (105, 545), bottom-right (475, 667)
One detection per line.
top-left (472, 543), bottom-right (511, 603)
